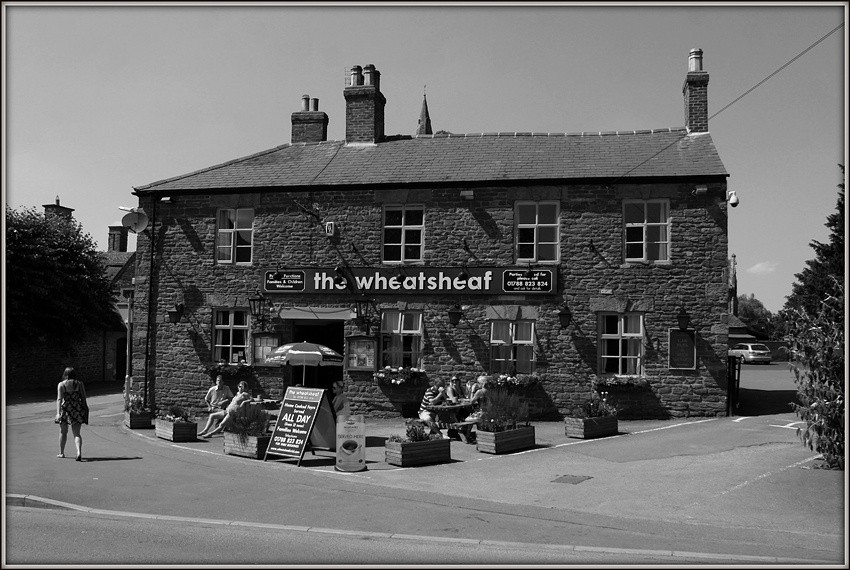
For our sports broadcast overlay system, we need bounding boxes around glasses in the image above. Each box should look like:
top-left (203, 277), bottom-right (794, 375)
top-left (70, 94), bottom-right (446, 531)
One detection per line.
top-left (451, 379), bottom-right (459, 382)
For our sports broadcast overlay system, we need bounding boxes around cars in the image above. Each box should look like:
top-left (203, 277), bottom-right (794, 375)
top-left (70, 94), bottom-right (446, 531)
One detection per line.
top-left (728, 342), bottom-right (772, 364)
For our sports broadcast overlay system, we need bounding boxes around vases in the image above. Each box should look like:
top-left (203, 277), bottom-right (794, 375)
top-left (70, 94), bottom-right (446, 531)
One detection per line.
top-left (565, 416), bottom-right (619, 438)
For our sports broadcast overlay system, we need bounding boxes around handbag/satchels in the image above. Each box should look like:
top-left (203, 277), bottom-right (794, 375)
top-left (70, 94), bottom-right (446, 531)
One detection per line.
top-left (84, 405), bottom-right (89, 424)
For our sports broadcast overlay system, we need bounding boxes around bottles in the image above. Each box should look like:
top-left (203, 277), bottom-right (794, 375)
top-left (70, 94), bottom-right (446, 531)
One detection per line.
top-left (468, 390), bottom-right (475, 399)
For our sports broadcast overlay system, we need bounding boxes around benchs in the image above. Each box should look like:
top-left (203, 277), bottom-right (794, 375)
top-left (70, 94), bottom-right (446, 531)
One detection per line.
top-left (453, 422), bottom-right (481, 443)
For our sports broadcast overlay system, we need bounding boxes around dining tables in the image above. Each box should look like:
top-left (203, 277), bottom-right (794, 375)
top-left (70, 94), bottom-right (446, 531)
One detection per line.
top-left (246, 398), bottom-right (278, 404)
top-left (431, 396), bottom-right (474, 421)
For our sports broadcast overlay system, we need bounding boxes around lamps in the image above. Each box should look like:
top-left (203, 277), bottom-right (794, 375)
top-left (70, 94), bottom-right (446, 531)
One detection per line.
top-left (459, 190), bottom-right (474, 201)
top-left (677, 309), bottom-right (690, 330)
top-left (166, 306), bottom-right (185, 325)
top-left (249, 290), bottom-right (273, 331)
top-left (557, 305), bottom-right (573, 330)
top-left (448, 304), bottom-right (466, 326)
top-left (351, 293), bottom-right (375, 330)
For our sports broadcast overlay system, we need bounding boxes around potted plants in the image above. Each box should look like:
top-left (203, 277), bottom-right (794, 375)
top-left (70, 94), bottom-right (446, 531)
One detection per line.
top-left (153, 407), bottom-right (198, 441)
top-left (125, 399), bottom-right (150, 428)
top-left (224, 406), bottom-right (269, 459)
top-left (477, 386), bottom-right (536, 453)
top-left (386, 420), bottom-right (450, 465)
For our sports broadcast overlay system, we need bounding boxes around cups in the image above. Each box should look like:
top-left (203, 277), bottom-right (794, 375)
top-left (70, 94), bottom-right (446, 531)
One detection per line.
top-left (342, 439), bottom-right (359, 455)
top-left (452, 397), bottom-right (458, 403)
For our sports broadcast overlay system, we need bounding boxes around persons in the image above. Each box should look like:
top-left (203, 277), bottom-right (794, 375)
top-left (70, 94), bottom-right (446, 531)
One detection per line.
top-left (418, 374), bottom-right (490, 443)
top-left (205, 375), bottom-right (234, 433)
top-left (55, 368), bottom-right (88, 461)
top-left (197, 381), bottom-right (254, 437)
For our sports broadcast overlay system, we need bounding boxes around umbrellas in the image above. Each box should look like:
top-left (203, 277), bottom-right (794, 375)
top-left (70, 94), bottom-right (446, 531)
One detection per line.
top-left (265, 341), bottom-right (344, 387)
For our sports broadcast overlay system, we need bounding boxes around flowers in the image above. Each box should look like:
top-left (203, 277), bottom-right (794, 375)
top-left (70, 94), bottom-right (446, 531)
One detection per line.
top-left (580, 392), bottom-right (616, 413)
top-left (204, 359), bottom-right (249, 378)
top-left (371, 365), bottom-right (419, 387)
top-left (492, 371), bottom-right (519, 388)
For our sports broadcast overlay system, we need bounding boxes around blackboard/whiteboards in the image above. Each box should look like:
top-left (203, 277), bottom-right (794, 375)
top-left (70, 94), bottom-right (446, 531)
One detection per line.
top-left (266, 387), bottom-right (339, 459)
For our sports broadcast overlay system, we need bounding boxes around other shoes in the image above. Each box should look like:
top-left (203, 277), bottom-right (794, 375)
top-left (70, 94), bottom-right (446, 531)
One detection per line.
top-left (75, 455), bottom-right (81, 461)
top-left (57, 454), bottom-right (64, 458)
